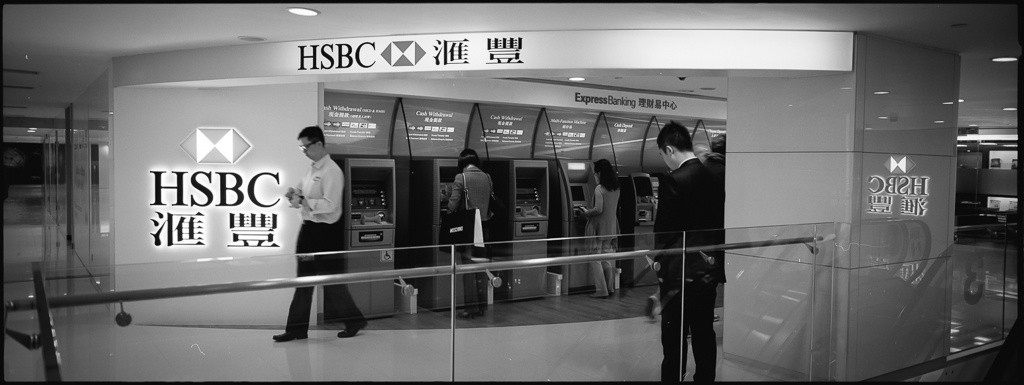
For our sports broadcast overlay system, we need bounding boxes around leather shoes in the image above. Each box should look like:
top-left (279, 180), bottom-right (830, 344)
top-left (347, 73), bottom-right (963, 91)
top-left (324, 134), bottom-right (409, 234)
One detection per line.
top-left (273, 332), bottom-right (308, 341)
top-left (337, 320), bottom-right (367, 337)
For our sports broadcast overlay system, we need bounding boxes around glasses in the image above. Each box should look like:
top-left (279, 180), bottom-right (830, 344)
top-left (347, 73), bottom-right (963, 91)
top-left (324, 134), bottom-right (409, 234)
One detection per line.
top-left (298, 141), bottom-right (317, 149)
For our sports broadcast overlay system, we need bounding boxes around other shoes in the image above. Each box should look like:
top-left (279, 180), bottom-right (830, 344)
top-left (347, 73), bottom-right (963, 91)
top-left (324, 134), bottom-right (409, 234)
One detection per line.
top-left (590, 293), bottom-right (608, 298)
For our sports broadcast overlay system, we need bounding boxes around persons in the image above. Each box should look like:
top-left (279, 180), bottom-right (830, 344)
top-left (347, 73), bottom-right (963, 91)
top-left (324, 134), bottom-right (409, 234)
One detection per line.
top-left (449, 148), bottom-right (494, 319)
top-left (655, 117), bottom-right (728, 382)
top-left (271, 127), bottom-right (367, 341)
top-left (582, 158), bottom-right (620, 297)
top-left (647, 132), bottom-right (726, 339)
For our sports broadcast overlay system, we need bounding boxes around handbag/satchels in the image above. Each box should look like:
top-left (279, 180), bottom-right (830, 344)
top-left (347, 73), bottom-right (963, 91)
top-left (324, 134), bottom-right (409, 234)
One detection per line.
top-left (438, 208), bottom-right (485, 253)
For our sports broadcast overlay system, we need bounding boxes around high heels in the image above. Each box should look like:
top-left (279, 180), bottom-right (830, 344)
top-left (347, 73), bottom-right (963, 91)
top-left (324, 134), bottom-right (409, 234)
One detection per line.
top-left (459, 311), bottom-right (475, 319)
top-left (476, 308), bottom-right (485, 316)
